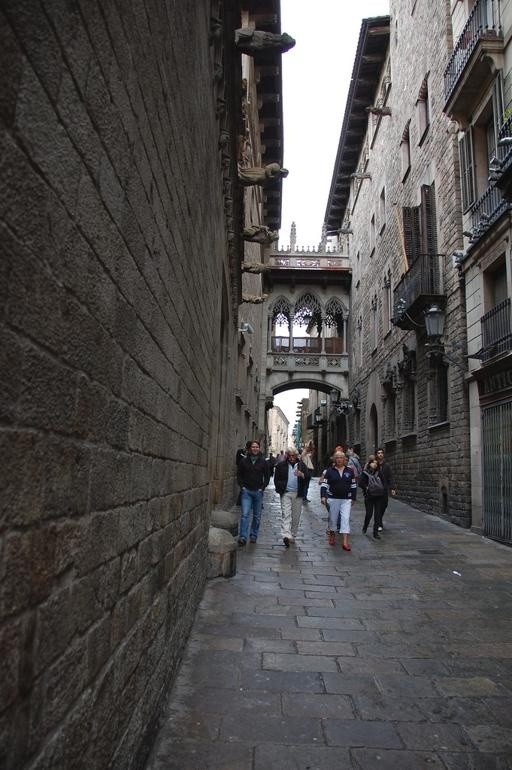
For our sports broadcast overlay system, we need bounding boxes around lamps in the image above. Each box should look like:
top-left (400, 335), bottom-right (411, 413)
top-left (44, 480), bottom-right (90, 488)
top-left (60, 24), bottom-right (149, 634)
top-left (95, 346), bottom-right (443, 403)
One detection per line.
top-left (421, 302), bottom-right (479, 373)
top-left (316, 410), bottom-right (328, 427)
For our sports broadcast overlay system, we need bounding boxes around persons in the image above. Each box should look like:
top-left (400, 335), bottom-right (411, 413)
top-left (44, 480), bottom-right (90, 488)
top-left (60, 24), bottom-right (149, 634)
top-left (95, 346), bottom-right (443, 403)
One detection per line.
top-left (244, 224), bottom-right (279, 247)
top-left (320, 450), bottom-right (358, 551)
top-left (336, 228), bottom-right (353, 235)
top-left (239, 160), bottom-right (289, 188)
top-left (349, 171), bottom-right (372, 179)
top-left (243, 261), bottom-right (273, 277)
top-left (274, 446), bottom-right (311, 547)
top-left (359, 458), bottom-right (388, 541)
top-left (242, 291), bottom-right (268, 305)
top-left (365, 107), bottom-right (390, 115)
top-left (374, 447), bottom-right (397, 532)
top-left (268, 437), bottom-right (376, 534)
top-left (236, 441), bottom-right (265, 510)
top-left (236, 441), bottom-right (270, 545)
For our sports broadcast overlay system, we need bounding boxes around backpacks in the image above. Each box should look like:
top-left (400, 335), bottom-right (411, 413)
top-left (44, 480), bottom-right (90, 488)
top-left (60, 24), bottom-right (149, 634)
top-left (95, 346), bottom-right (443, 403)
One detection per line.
top-left (362, 471), bottom-right (384, 497)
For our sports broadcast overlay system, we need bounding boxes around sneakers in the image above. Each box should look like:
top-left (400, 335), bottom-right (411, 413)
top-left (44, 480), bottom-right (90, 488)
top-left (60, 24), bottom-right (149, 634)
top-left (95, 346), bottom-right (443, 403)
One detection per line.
top-left (283, 537), bottom-right (295, 548)
top-left (326, 528), bottom-right (351, 551)
top-left (362, 526), bottom-right (383, 539)
top-left (237, 538), bottom-right (256, 545)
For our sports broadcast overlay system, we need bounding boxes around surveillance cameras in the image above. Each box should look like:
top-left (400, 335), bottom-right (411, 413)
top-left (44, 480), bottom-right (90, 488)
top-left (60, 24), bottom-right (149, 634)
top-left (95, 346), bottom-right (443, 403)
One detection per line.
top-left (244, 322), bottom-right (254, 334)
